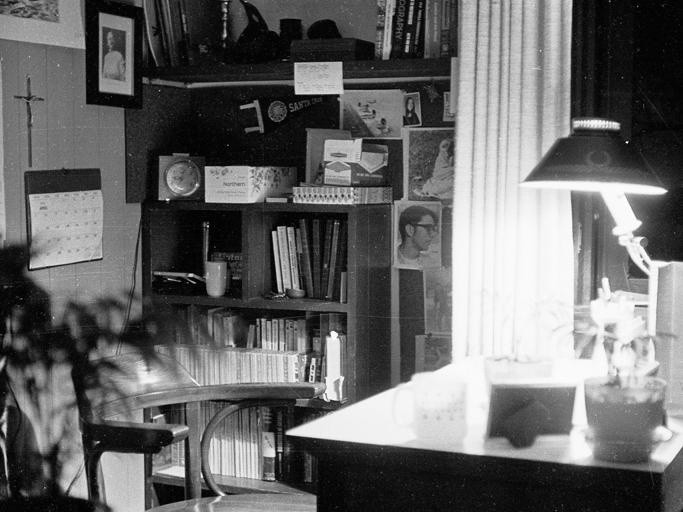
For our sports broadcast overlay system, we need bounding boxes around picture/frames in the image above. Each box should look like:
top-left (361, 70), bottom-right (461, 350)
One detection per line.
top-left (85, 0), bottom-right (143, 110)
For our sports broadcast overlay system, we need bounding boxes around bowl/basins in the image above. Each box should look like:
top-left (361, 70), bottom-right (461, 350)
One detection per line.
top-left (286, 289), bottom-right (306, 299)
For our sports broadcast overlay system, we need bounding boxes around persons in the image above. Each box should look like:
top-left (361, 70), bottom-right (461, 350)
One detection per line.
top-left (102, 29), bottom-right (126, 80)
top-left (358, 101), bottom-right (392, 134)
top-left (402, 97), bottom-right (420, 125)
top-left (397, 206), bottom-right (439, 268)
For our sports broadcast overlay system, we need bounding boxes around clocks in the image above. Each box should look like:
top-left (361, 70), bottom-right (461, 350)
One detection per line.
top-left (158, 155), bottom-right (204, 202)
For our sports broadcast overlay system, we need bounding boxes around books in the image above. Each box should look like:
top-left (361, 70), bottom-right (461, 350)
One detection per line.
top-left (154, 307), bottom-right (347, 387)
top-left (372, 0), bottom-right (458, 70)
top-left (272, 217), bottom-right (348, 301)
top-left (171, 400), bottom-right (327, 484)
top-left (144, 0), bottom-right (231, 74)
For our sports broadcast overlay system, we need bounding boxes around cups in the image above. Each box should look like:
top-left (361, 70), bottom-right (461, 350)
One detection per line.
top-left (584, 376), bottom-right (667, 461)
top-left (201, 259), bottom-right (229, 299)
top-left (279, 17), bottom-right (301, 56)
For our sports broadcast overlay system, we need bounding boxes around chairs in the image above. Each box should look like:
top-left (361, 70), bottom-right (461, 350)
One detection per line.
top-left (70, 353), bottom-right (327, 512)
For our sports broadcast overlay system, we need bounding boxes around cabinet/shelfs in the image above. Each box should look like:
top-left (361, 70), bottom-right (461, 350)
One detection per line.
top-left (138, 205), bottom-right (359, 494)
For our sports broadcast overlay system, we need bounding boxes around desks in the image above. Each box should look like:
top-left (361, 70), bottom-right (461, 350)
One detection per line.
top-left (284, 294), bottom-right (683, 512)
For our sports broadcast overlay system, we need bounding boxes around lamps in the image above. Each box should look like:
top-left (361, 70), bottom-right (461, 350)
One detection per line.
top-left (518, 120), bottom-right (668, 278)
